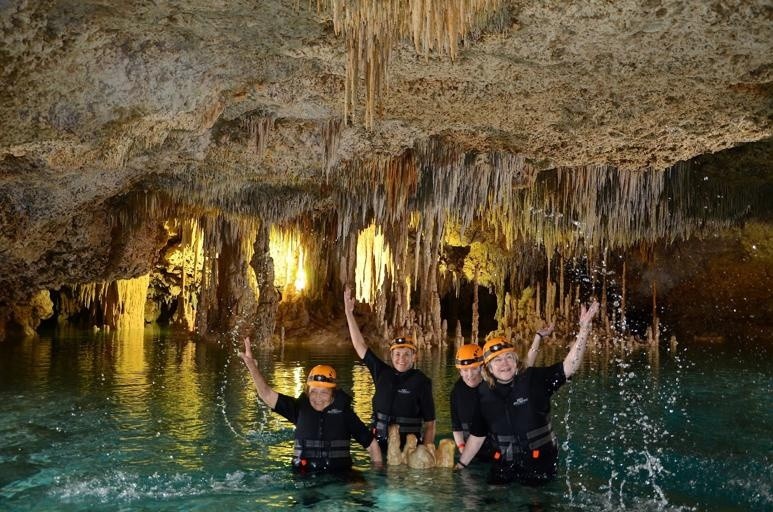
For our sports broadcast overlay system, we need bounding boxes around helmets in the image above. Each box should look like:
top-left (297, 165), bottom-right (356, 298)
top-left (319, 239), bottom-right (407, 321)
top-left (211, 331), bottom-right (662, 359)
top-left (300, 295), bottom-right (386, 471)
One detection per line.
top-left (389, 336), bottom-right (417, 351)
top-left (455, 337), bottom-right (515, 368)
top-left (306, 365), bottom-right (337, 388)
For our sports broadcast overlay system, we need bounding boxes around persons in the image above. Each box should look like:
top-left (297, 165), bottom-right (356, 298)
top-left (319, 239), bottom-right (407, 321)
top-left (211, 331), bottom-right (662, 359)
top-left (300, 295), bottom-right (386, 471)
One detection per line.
top-left (343, 286), bottom-right (436, 456)
top-left (453, 298), bottom-right (600, 487)
top-left (450, 323), bottom-right (555, 463)
top-left (240, 336), bottom-right (383, 475)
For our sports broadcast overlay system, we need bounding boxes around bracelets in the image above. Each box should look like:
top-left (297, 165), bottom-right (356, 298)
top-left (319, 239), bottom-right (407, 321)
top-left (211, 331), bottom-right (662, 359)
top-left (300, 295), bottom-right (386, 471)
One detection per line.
top-left (536, 333), bottom-right (543, 338)
top-left (457, 461), bottom-right (467, 467)
top-left (457, 444), bottom-right (465, 448)
top-left (529, 347), bottom-right (539, 352)
top-left (579, 321), bottom-right (592, 327)
